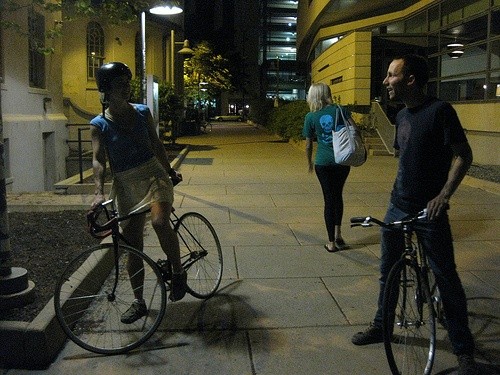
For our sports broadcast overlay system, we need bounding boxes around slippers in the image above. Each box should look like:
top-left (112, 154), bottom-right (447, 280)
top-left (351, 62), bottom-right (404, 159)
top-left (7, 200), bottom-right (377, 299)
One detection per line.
top-left (323, 244), bottom-right (336, 252)
top-left (335, 238), bottom-right (344, 243)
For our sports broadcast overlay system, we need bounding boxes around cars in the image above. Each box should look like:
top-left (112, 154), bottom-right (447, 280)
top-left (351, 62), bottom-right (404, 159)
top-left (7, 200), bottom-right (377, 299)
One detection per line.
top-left (213, 112), bottom-right (244, 122)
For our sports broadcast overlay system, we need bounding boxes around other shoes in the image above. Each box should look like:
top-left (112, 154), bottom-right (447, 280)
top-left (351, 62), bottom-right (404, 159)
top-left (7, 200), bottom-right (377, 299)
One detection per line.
top-left (457, 353), bottom-right (476, 375)
top-left (351, 322), bottom-right (393, 346)
top-left (169, 271), bottom-right (188, 302)
top-left (121, 299), bottom-right (148, 324)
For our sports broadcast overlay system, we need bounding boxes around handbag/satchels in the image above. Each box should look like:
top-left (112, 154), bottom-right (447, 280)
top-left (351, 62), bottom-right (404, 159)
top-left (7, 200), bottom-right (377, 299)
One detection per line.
top-left (332, 101), bottom-right (366, 167)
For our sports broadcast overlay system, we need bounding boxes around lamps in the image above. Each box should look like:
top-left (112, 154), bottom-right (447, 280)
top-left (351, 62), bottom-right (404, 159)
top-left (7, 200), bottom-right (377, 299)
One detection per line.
top-left (446, 38), bottom-right (465, 59)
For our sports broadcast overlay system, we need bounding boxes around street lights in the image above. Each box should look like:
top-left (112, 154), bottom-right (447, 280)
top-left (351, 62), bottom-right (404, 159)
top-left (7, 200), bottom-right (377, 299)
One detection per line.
top-left (140, 4), bottom-right (184, 106)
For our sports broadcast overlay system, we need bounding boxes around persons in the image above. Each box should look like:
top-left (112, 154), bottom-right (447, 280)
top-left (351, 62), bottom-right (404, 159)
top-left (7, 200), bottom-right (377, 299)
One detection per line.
top-left (352, 53), bottom-right (477, 375)
top-left (302, 82), bottom-right (358, 253)
top-left (89, 62), bottom-right (188, 323)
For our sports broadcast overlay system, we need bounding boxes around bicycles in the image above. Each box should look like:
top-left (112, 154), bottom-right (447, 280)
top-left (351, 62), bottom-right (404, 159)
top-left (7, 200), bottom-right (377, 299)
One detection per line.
top-left (351, 208), bottom-right (451, 374)
top-left (53, 172), bottom-right (224, 356)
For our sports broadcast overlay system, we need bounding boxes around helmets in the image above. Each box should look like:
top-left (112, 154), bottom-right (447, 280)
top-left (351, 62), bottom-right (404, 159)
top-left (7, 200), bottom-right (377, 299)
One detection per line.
top-left (96, 62), bottom-right (133, 92)
